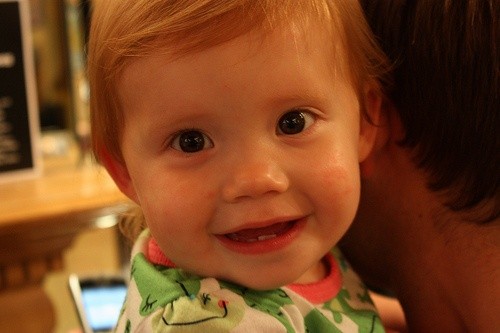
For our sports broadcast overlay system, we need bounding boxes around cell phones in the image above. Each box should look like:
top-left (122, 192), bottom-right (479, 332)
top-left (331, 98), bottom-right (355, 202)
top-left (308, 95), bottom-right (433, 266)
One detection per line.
top-left (70, 272), bottom-right (130, 332)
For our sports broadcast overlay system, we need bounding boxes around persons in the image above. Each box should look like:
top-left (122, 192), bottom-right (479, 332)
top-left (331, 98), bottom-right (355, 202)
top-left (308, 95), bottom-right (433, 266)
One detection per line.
top-left (82, 1), bottom-right (413, 333)
top-left (331, 0), bottom-right (500, 333)
